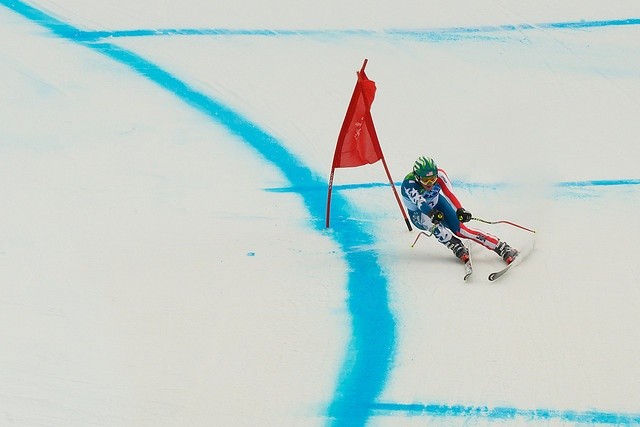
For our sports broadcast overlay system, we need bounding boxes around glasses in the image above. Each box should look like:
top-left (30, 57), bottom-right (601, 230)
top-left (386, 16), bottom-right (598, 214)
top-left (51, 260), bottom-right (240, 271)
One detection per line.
top-left (414, 172), bottom-right (438, 186)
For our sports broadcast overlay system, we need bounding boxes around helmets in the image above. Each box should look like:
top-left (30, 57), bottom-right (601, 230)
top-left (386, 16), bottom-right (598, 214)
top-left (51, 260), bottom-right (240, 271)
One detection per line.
top-left (413, 155), bottom-right (438, 179)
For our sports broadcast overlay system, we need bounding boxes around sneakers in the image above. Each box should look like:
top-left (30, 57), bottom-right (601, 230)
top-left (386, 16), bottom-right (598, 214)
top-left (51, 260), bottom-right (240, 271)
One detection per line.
top-left (494, 241), bottom-right (518, 264)
top-left (441, 235), bottom-right (469, 262)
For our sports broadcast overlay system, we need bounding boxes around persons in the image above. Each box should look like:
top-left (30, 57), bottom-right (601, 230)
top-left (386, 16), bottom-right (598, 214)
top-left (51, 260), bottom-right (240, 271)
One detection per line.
top-left (401, 154), bottom-right (519, 263)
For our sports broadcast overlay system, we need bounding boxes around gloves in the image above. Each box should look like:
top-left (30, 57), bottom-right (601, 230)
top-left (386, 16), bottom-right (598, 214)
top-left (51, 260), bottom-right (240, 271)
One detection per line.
top-left (456, 208), bottom-right (472, 223)
top-left (428, 210), bottom-right (444, 225)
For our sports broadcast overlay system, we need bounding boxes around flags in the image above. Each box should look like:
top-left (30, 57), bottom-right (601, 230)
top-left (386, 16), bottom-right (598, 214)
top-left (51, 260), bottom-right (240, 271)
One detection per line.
top-left (332, 69), bottom-right (383, 168)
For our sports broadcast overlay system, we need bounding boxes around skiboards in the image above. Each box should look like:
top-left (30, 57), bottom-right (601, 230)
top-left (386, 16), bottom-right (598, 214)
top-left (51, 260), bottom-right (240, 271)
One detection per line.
top-left (464, 240), bottom-right (529, 281)
